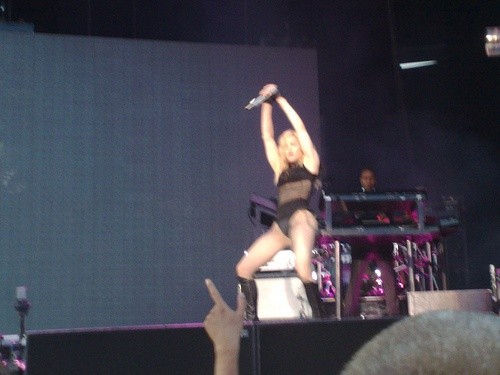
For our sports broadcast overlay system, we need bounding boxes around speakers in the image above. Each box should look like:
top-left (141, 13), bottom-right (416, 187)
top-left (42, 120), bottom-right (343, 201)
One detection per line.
top-left (257, 315), bottom-right (408, 375)
top-left (26, 320), bottom-right (257, 375)
top-left (401, 290), bottom-right (493, 318)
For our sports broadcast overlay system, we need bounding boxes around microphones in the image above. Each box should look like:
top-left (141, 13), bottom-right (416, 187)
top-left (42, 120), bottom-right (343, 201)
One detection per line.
top-left (15, 286), bottom-right (30, 313)
top-left (245, 89), bottom-right (279, 111)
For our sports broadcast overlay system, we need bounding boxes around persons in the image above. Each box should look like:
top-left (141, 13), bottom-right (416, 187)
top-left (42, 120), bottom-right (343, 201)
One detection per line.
top-left (343, 169), bottom-right (402, 320)
top-left (203, 278), bottom-right (500, 375)
top-left (236, 84), bottom-right (325, 321)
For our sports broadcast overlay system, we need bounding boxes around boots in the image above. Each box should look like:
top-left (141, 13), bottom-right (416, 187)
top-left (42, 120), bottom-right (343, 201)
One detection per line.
top-left (235, 275), bottom-right (260, 321)
top-left (303, 282), bottom-right (325, 319)
